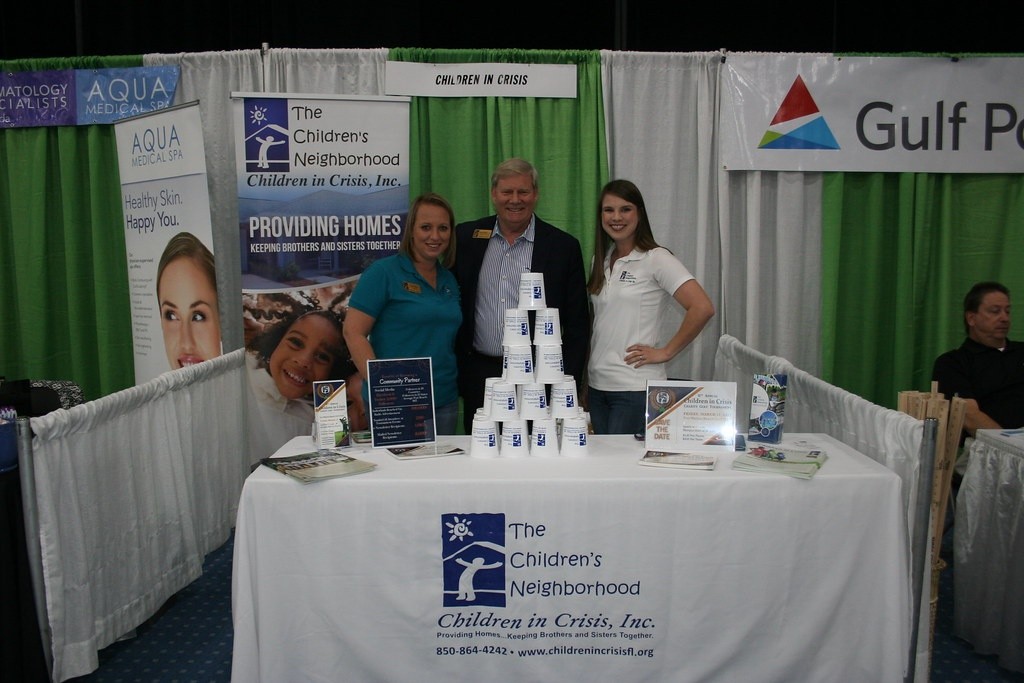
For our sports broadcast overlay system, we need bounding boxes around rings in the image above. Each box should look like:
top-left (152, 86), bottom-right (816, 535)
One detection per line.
top-left (640, 355), bottom-right (643, 361)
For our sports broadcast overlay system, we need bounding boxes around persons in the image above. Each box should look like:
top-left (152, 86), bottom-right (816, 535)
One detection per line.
top-left (343, 191), bottom-right (463, 435)
top-left (932, 280), bottom-right (1024, 474)
top-left (442, 159), bottom-right (592, 435)
top-left (581, 180), bottom-right (715, 434)
top-left (156, 232), bottom-right (222, 372)
top-left (241, 280), bottom-right (369, 435)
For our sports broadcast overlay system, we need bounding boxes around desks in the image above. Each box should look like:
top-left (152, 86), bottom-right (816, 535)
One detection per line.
top-left (230, 435), bottom-right (914, 683)
top-left (952, 430), bottom-right (1024, 672)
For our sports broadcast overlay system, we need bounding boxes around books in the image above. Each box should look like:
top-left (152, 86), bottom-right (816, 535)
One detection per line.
top-left (976, 426), bottom-right (1024, 450)
top-left (639, 450), bottom-right (717, 471)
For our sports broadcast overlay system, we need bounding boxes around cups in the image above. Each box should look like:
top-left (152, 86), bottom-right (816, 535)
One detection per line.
top-left (472, 274), bottom-right (590, 460)
